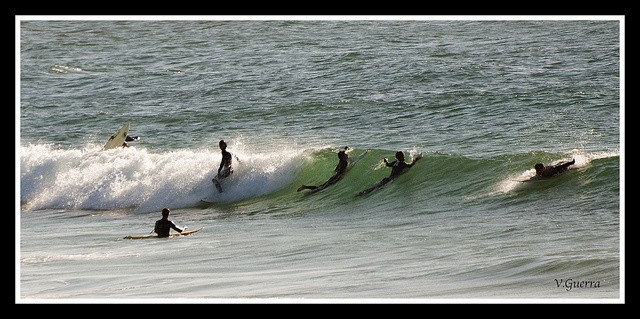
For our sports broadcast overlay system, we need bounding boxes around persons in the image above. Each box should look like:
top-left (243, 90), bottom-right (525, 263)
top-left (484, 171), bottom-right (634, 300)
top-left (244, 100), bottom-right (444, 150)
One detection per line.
top-left (297, 146), bottom-right (350, 193)
top-left (357, 151), bottom-right (423, 198)
top-left (154, 208), bottom-right (188, 237)
top-left (212, 139), bottom-right (240, 194)
top-left (529, 159), bottom-right (576, 179)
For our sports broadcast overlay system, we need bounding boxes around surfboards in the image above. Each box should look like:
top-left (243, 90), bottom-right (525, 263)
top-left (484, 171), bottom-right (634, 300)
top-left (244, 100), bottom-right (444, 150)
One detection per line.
top-left (511, 177), bottom-right (554, 183)
top-left (123, 227), bottom-right (202, 238)
top-left (105, 123), bottom-right (132, 149)
top-left (201, 199), bottom-right (216, 204)
